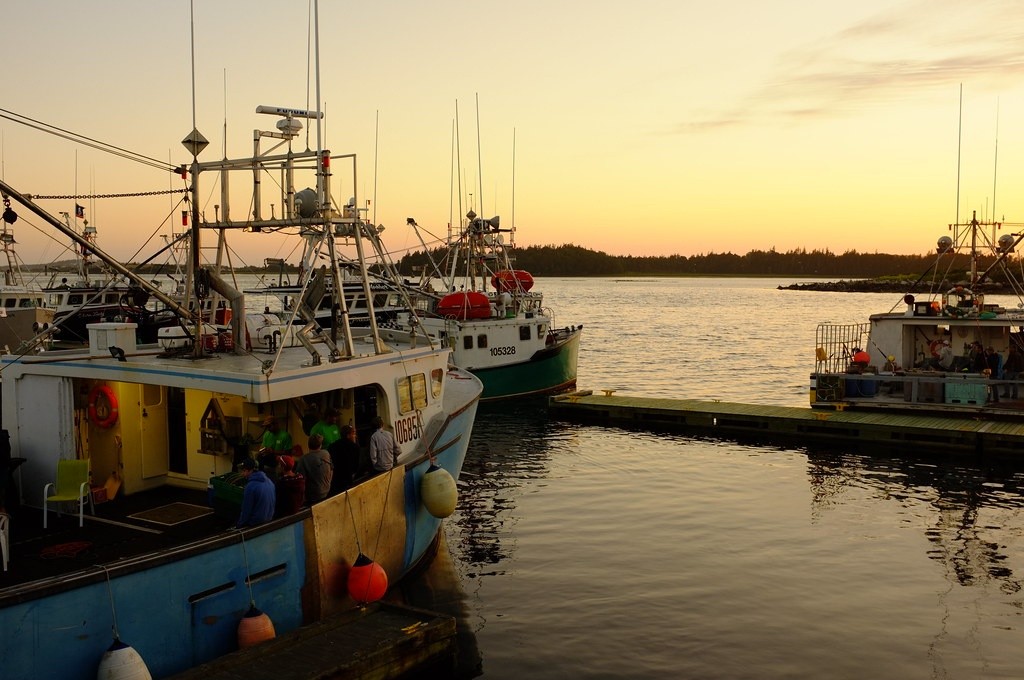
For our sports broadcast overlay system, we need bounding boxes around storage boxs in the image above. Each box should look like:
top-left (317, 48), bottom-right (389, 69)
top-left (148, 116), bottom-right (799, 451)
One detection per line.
top-left (92, 486), bottom-right (108, 504)
top-left (245, 314), bottom-right (314, 348)
top-left (209, 474), bottom-right (245, 514)
top-left (86, 322), bottom-right (139, 355)
top-left (818, 364), bottom-right (991, 407)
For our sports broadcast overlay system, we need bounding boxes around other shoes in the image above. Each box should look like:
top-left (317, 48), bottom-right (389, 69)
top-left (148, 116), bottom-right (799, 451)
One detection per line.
top-left (1000, 392), bottom-right (1010, 398)
top-left (993, 400), bottom-right (999, 403)
top-left (1011, 396), bottom-right (1018, 399)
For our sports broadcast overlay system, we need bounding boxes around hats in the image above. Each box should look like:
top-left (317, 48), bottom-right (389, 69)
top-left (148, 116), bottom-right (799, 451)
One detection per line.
top-left (943, 340), bottom-right (949, 345)
top-left (276, 455), bottom-right (294, 468)
top-left (233, 455), bottom-right (255, 469)
top-left (971, 341), bottom-right (979, 344)
top-left (324, 405), bottom-right (340, 417)
top-left (985, 346), bottom-right (994, 351)
top-left (262, 415), bottom-right (279, 425)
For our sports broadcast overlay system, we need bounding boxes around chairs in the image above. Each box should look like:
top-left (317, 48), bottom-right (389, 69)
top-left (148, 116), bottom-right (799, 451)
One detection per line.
top-left (43, 458), bottom-right (95, 527)
top-left (0, 508), bottom-right (10, 571)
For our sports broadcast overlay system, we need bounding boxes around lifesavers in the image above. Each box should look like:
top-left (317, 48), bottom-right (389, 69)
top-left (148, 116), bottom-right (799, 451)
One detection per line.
top-left (930, 339), bottom-right (945, 356)
top-left (87, 384), bottom-right (119, 429)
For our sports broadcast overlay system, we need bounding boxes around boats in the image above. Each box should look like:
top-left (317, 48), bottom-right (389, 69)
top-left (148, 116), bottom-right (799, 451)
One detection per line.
top-left (807, 210), bottom-right (1024, 421)
top-left (0, 104), bottom-right (585, 679)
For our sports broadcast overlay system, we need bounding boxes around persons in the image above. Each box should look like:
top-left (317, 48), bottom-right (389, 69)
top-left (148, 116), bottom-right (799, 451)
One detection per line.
top-left (298, 434), bottom-right (333, 505)
top-left (968, 342), bottom-right (1002, 408)
top-left (236, 460), bottom-right (276, 527)
top-left (257, 416), bottom-right (293, 482)
top-left (273, 455), bottom-right (305, 519)
top-left (938, 340), bottom-right (953, 371)
top-left (368, 415), bottom-right (401, 474)
top-left (327, 426), bottom-right (367, 491)
top-left (309, 407), bottom-right (341, 449)
